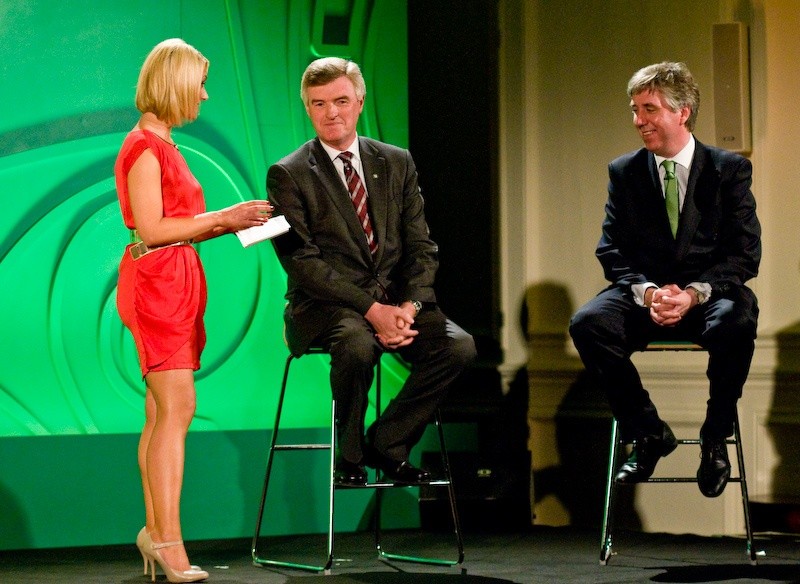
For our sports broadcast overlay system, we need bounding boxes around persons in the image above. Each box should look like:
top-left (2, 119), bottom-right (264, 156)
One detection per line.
top-left (568, 61), bottom-right (765, 496)
top-left (112, 37), bottom-right (279, 582)
top-left (264, 54), bottom-right (481, 488)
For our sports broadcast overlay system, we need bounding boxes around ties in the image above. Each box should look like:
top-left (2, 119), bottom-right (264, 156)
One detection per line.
top-left (338, 151), bottom-right (377, 263)
top-left (662, 160), bottom-right (679, 238)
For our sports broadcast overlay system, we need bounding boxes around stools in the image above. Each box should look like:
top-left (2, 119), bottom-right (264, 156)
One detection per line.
top-left (594, 335), bottom-right (759, 569)
top-left (249, 335), bottom-right (462, 574)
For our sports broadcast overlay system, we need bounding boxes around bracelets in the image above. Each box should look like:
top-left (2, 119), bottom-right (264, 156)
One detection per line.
top-left (692, 286), bottom-right (706, 304)
top-left (409, 299), bottom-right (423, 318)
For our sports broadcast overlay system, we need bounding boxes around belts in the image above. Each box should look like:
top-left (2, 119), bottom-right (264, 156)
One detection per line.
top-left (129, 229), bottom-right (196, 261)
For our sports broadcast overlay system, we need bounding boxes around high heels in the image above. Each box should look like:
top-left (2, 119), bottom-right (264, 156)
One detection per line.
top-left (143, 533), bottom-right (210, 583)
top-left (135, 526), bottom-right (202, 577)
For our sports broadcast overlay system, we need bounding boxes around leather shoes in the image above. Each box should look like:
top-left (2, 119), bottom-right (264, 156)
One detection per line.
top-left (335, 467), bottom-right (368, 487)
top-left (697, 425), bottom-right (731, 498)
top-left (614, 422), bottom-right (678, 484)
top-left (363, 438), bottom-right (432, 484)
